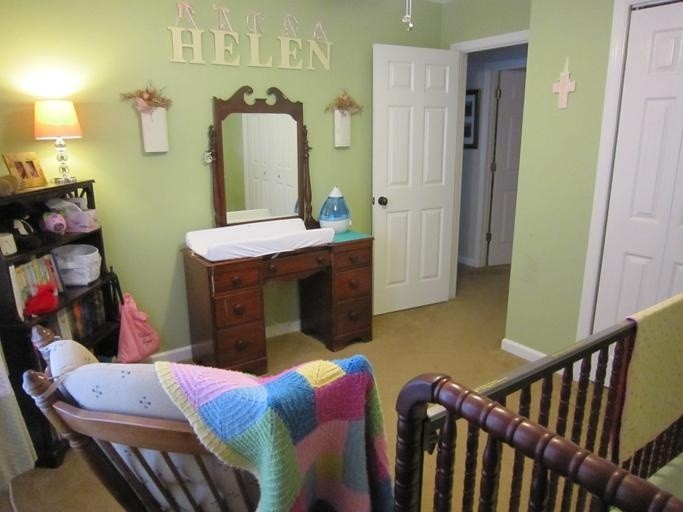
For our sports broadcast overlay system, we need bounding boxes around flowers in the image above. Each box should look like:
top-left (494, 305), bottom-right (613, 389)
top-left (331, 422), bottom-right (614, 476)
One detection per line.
top-left (120, 83), bottom-right (172, 114)
top-left (324, 91), bottom-right (364, 114)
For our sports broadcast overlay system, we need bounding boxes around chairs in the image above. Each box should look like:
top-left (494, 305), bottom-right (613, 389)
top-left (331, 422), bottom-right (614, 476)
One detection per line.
top-left (21, 325), bottom-right (365, 511)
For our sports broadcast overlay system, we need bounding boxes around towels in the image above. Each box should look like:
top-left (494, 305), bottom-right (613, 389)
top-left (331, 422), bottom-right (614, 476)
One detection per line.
top-left (613, 295), bottom-right (683, 463)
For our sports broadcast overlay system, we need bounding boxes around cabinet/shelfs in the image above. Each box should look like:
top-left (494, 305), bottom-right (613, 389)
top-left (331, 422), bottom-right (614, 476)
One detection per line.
top-left (0, 178), bottom-right (124, 469)
top-left (241, 114), bottom-right (299, 218)
top-left (573, 2), bottom-right (683, 389)
top-left (182, 226), bottom-right (374, 377)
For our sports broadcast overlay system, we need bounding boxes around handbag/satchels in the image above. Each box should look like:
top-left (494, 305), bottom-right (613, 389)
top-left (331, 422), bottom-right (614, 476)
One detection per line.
top-left (111, 273), bottom-right (159, 364)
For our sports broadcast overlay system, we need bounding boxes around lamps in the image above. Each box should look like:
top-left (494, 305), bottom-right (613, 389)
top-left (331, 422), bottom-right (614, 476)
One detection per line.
top-left (34, 101), bottom-right (81, 185)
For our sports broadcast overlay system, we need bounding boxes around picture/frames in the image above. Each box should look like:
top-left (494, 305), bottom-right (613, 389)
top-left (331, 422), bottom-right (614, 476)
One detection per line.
top-left (462, 89), bottom-right (481, 149)
top-left (1, 151), bottom-right (47, 191)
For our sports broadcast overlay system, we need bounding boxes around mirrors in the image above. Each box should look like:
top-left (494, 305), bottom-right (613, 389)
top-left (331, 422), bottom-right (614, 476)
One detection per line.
top-left (212, 87), bottom-right (312, 230)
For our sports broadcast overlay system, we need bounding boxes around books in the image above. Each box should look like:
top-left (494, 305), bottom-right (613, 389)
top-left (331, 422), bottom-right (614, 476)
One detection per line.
top-left (54, 289), bottom-right (110, 347)
top-left (7, 251), bottom-right (61, 321)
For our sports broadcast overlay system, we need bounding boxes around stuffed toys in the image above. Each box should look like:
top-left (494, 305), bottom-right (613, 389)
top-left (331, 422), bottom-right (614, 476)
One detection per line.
top-left (40, 210), bottom-right (67, 236)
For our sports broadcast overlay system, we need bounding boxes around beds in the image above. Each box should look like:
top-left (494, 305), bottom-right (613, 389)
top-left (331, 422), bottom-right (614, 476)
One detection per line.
top-left (392, 294), bottom-right (683, 510)
